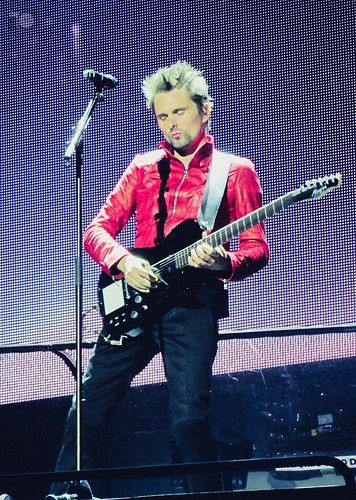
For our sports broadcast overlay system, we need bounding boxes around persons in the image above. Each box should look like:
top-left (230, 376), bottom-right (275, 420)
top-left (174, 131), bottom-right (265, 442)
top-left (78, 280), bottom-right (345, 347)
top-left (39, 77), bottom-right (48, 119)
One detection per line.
top-left (45, 59), bottom-right (270, 500)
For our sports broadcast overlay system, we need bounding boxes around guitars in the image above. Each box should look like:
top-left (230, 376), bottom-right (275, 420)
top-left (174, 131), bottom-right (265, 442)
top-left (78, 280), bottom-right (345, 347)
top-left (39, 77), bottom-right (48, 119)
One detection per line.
top-left (96, 170), bottom-right (344, 345)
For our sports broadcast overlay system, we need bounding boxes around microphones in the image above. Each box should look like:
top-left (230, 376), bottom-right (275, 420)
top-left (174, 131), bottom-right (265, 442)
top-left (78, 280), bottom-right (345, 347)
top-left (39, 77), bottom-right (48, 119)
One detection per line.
top-left (83, 69), bottom-right (118, 90)
